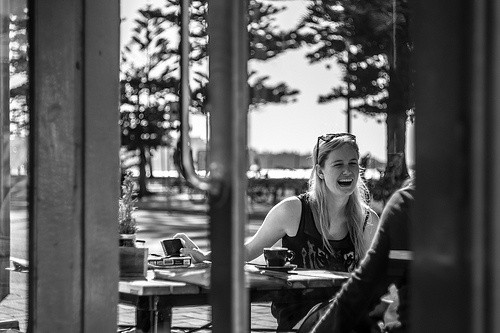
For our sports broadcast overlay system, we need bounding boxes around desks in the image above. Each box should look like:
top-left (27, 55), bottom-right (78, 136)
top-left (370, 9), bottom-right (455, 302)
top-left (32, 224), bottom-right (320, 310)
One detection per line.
top-left (111, 264), bottom-right (368, 333)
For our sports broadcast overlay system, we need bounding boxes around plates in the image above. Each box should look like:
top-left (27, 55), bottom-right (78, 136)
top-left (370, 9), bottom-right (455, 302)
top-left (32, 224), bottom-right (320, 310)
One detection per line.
top-left (255, 264), bottom-right (297, 272)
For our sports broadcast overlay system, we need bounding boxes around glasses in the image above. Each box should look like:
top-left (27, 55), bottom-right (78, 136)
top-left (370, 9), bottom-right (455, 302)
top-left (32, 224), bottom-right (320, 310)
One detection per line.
top-left (316, 133), bottom-right (356, 164)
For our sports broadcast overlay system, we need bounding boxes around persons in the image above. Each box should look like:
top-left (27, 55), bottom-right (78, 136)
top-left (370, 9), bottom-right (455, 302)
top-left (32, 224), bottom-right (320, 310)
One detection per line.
top-left (172, 134), bottom-right (381, 333)
top-left (307, 177), bottom-right (414, 333)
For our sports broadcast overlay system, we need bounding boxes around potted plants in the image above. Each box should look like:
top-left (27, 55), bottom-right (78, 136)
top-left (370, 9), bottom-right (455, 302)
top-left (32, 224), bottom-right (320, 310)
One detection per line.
top-left (115, 174), bottom-right (149, 280)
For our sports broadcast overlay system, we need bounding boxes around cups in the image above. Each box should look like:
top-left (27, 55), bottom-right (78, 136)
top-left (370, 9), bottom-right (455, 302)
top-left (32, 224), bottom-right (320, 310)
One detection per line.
top-left (264, 248), bottom-right (295, 267)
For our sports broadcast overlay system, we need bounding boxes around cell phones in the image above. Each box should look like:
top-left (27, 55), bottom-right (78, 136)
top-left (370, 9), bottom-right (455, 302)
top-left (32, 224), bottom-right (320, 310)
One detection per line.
top-left (161, 238), bottom-right (185, 258)
